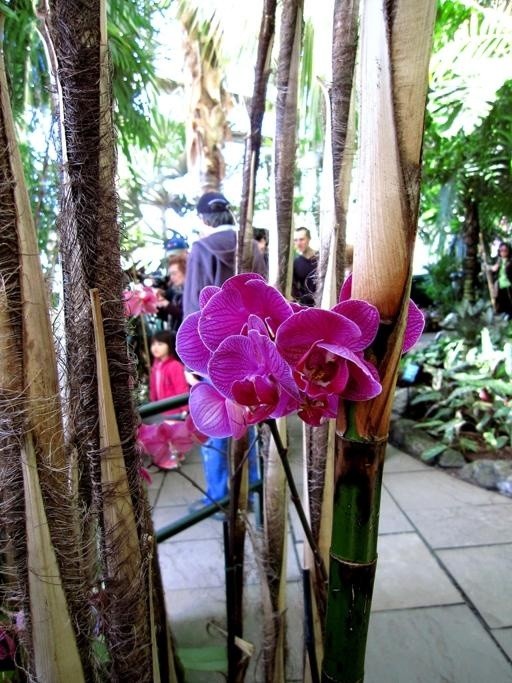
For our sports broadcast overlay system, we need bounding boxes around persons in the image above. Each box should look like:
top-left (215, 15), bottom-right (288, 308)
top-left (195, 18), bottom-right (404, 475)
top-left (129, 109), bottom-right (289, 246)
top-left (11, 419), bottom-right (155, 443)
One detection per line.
top-left (149, 331), bottom-right (191, 421)
top-left (410, 274), bottom-right (442, 334)
top-left (181, 190), bottom-right (268, 518)
top-left (489, 242), bottom-right (511, 319)
top-left (146, 225), bottom-right (320, 330)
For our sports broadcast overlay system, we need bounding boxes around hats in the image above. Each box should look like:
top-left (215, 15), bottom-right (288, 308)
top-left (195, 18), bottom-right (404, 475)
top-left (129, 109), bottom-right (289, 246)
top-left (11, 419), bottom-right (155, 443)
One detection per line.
top-left (165, 238), bottom-right (190, 250)
top-left (196, 192), bottom-right (230, 216)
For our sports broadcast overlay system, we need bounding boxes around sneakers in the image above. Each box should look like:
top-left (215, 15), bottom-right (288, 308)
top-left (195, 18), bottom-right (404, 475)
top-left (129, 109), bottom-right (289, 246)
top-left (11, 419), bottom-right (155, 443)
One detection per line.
top-left (187, 499), bottom-right (226, 521)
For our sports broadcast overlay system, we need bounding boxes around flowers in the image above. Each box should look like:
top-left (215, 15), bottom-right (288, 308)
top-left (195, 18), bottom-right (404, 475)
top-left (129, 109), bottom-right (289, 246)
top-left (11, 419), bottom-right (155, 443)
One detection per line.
top-left (172, 269), bottom-right (428, 443)
top-left (130, 407), bottom-right (195, 478)
top-left (117, 273), bottom-right (168, 321)
